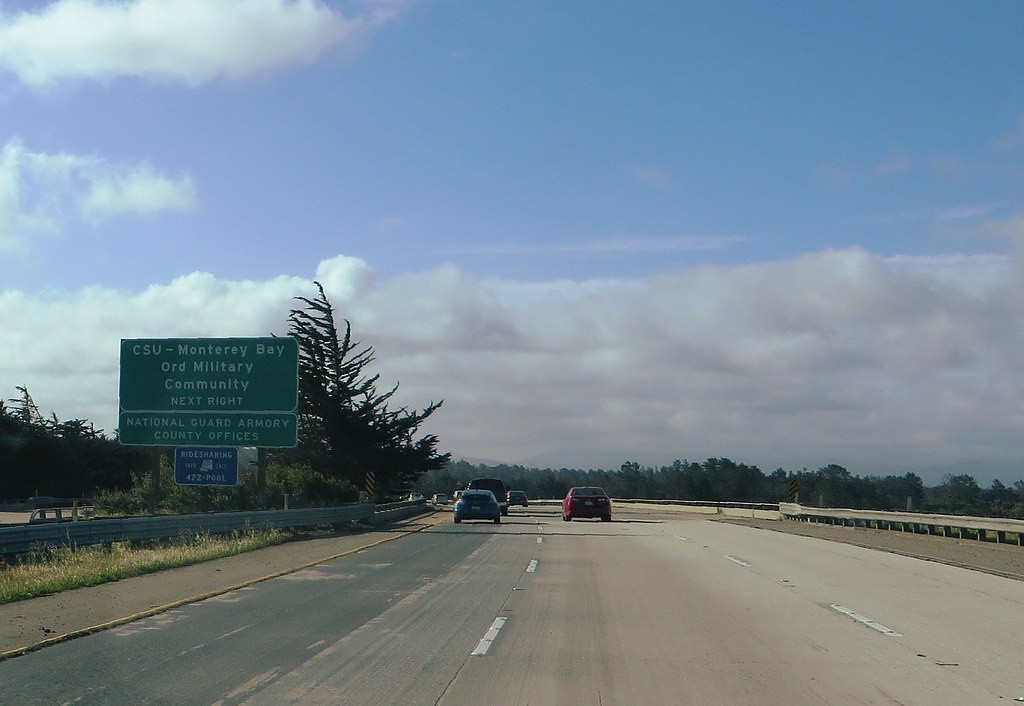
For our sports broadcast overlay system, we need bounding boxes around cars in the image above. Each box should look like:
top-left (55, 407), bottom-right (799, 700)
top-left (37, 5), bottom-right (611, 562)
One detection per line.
top-left (506, 491), bottom-right (528, 507)
top-left (431, 494), bottom-right (448, 505)
top-left (453, 491), bottom-right (501, 523)
top-left (561, 487), bottom-right (612, 522)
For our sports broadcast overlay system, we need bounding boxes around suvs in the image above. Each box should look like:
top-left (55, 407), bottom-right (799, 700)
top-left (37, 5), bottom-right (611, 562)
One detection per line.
top-left (410, 492), bottom-right (426, 502)
top-left (452, 491), bottom-right (463, 503)
top-left (465, 479), bottom-right (509, 516)
